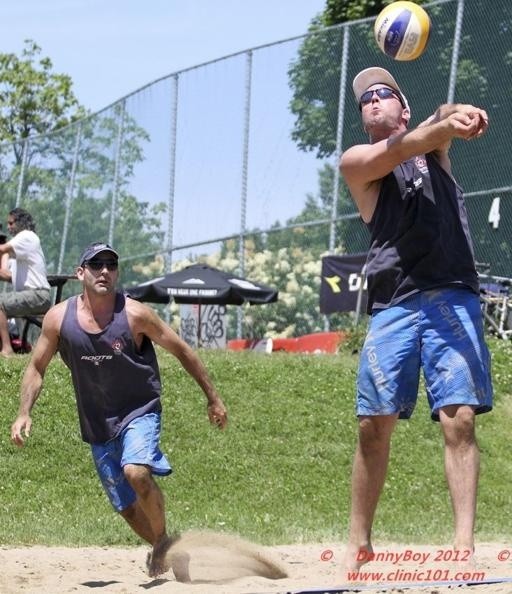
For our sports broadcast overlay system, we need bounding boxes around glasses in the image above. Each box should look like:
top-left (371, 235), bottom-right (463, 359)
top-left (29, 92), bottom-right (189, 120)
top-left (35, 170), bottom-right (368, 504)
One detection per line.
top-left (83, 260), bottom-right (119, 271)
top-left (358, 86), bottom-right (405, 109)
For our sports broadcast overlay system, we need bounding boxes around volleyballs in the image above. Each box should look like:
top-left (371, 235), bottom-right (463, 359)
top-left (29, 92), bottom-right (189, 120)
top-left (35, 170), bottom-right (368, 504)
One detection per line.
top-left (373, 1), bottom-right (432, 65)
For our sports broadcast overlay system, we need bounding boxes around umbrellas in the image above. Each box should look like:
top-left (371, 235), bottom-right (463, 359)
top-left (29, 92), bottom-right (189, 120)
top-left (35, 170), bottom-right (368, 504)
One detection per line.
top-left (118, 261), bottom-right (279, 351)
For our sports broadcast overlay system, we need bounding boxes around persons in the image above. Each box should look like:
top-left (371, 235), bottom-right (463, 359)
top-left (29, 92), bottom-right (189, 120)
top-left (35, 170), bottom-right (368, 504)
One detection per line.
top-left (0, 235), bottom-right (19, 341)
top-left (0, 208), bottom-right (51, 358)
top-left (11, 241), bottom-right (230, 583)
top-left (337, 66), bottom-right (490, 586)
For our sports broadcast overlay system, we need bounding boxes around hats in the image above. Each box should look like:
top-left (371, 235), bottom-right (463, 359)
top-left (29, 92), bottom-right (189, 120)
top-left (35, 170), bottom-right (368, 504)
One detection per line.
top-left (78, 241), bottom-right (119, 267)
top-left (352, 65), bottom-right (412, 125)
